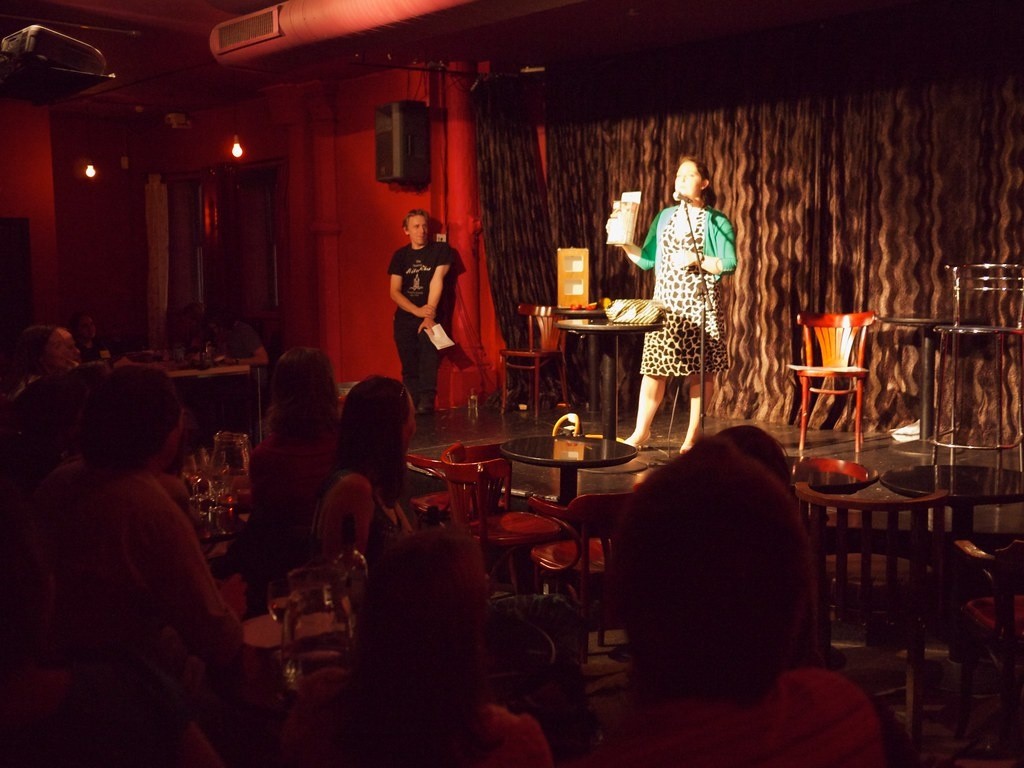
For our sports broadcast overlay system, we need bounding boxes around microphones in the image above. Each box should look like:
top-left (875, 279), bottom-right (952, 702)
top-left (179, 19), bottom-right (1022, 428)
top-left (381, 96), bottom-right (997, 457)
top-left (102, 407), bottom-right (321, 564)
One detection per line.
top-left (673, 191), bottom-right (692, 203)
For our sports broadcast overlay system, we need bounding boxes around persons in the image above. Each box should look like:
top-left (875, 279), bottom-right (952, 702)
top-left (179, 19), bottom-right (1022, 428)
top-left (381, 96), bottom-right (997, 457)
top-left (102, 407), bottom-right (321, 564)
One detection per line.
top-left (386, 210), bottom-right (461, 415)
top-left (0, 314), bottom-right (917, 768)
top-left (604, 155), bottom-right (737, 455)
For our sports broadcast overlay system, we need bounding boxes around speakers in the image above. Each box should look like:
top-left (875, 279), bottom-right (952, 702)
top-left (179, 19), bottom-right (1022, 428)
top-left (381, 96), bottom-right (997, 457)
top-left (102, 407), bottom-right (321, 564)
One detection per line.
top-left (374, 99), bottom-right (431, 181)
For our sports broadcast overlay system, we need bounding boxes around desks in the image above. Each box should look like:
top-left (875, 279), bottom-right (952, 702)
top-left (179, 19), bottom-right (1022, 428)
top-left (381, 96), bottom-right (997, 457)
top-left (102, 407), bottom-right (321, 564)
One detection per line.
top-left (500, 434), bottom-right (638, 594)
top-left (880, 312), bottom-right (985, 455)
top-left (172, 365), bottom-right (252, 444)
top-left (880, 463), bottom-right (1024, 657)
top-left (555, 318), bottom-right (665, 475)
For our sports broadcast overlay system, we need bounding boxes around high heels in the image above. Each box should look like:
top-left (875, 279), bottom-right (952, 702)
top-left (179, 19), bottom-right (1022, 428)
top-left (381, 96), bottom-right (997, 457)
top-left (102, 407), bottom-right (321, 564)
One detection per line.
top-left (623, 430), bottom-right (650, 449)
top-left (680, 432), bottom-right (702, 454)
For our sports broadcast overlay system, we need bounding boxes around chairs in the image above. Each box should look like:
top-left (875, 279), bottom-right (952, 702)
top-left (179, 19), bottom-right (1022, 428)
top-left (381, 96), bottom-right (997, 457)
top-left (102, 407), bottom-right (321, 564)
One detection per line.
top-left (928, 261), bottom-right (1024, 467)
top-left (500, 304), bottom-right (572, 416)
top-left (788, 312), bottom-right (876, 453)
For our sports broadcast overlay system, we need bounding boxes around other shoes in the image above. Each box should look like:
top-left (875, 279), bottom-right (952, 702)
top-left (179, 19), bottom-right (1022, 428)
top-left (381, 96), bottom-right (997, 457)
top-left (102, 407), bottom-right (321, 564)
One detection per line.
top-left (418, 402), bottom-right (433, 412)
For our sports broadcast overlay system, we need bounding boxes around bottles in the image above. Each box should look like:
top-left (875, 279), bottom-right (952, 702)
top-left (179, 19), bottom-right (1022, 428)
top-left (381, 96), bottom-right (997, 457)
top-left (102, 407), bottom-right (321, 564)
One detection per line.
top-left (282, 567), bottom-right (350, 682)
top-left (212, 430), bottom-right (250, 477)
top-left (467, 387), bottom-right (478, 417)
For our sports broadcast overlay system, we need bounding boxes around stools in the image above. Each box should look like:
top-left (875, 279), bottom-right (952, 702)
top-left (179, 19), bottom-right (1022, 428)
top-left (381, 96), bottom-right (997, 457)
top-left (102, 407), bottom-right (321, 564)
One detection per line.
top-left (787, 455), bottom-right (1024, 763)
top-left (280, 445), bottom-right (628, 686)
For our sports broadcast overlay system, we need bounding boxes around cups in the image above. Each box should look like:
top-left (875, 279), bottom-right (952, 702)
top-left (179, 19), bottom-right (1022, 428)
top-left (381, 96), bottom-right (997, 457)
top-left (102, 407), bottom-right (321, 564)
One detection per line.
top-left (188, 475), bottom-right (235, 535)
